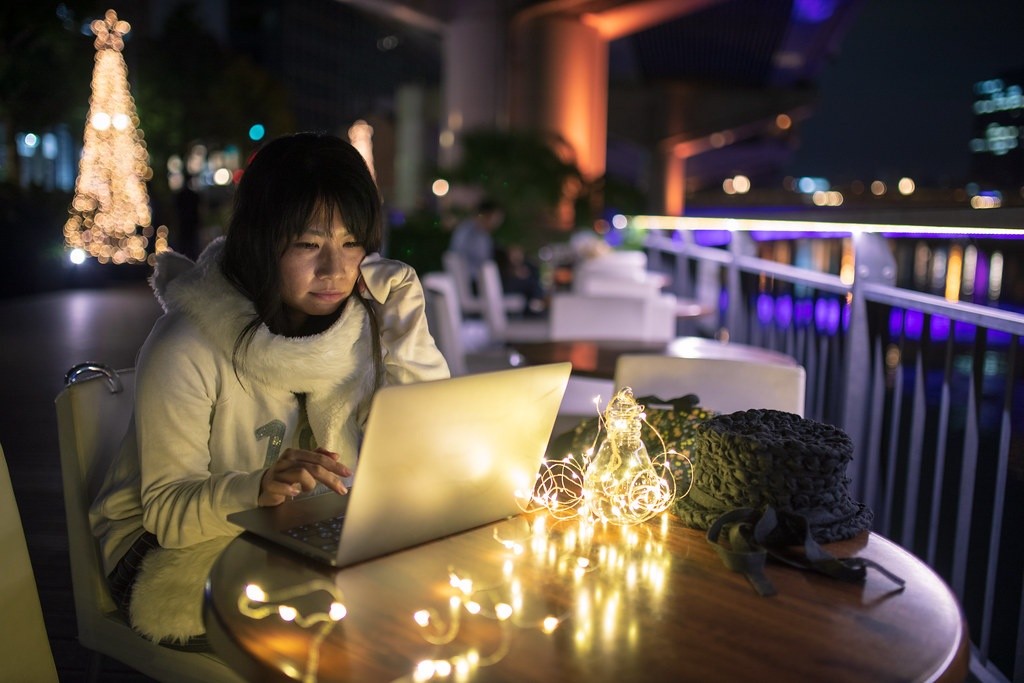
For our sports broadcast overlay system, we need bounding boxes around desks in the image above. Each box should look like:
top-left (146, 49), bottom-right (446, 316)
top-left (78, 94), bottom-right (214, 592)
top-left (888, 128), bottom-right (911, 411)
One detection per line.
top-left (530, 291), bottom-right (716, 320)
top-left (201, 530), bottom-right (971, 683)
top-left (506, 336), bottom-right (668, 383)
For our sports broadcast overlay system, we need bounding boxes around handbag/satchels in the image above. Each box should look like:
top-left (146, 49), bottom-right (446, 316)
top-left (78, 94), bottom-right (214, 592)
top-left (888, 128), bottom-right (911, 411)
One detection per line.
top-left (553, 392), bottom-right (722, 516)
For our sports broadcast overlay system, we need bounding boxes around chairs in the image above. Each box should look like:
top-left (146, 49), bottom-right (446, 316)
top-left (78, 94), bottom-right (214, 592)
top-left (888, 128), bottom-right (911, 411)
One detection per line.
top-left (55, 361), bottom-right (244, 683)
top-left (549, 295), bottom-right (676, 342)
top-left (0, 445), bottom-right (59, 683)
top-left (479, 261), bottom-right (550, 340)
top-left (424, 272), bottom-right (464, 337)
top-left (582, 277), bottom-right (661, 297)
top-left (424, 288), bottom-right (466, 377)
top-left (572, 250), bottom-right (648, 291)
top-left (613, 355), bottom-right (807, 418)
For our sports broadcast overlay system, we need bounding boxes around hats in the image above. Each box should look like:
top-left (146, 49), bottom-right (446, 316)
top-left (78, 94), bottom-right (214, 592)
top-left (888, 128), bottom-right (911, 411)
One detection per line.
top-left (666, 408), bottom-right (906, 597)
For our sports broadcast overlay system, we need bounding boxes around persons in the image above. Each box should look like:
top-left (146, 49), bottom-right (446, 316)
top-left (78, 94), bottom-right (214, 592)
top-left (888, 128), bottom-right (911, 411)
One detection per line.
top-left (89, 132), bottom-right (451, 683)
top-left (450, 196), bottom-right (550, 320)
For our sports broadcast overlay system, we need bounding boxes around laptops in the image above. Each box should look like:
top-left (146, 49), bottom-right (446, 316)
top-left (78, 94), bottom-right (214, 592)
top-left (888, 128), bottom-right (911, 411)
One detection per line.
top-left (226, 362), bottom-right (573, 569)
